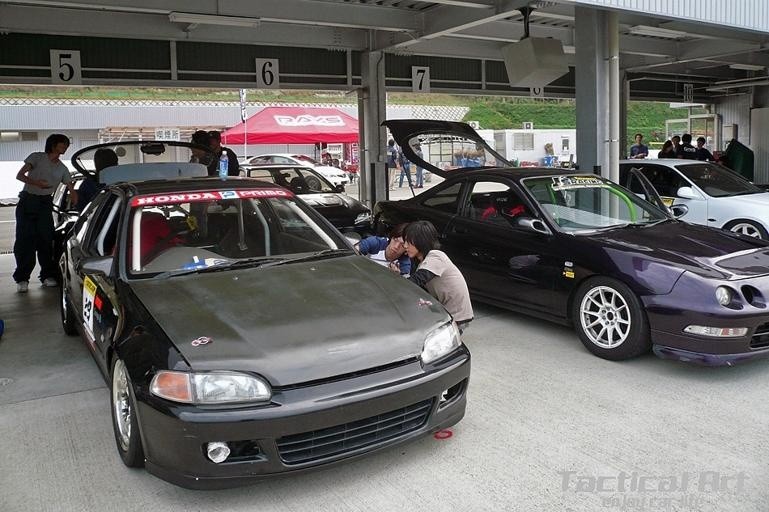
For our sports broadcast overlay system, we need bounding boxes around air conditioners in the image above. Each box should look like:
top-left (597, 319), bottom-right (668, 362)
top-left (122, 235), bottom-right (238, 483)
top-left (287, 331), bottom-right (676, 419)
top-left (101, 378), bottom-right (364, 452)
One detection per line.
top-left (522, 122), bottom-right (533, 129)
top-left (467, 121), bottom-right (479, 129)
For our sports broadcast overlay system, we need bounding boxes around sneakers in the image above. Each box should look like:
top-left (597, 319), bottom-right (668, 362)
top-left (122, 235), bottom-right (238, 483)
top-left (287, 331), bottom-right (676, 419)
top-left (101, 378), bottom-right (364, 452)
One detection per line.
top-left (42, 277), bottom-right (57, 287)
top-left (17, 282), bottom-right (28, 291)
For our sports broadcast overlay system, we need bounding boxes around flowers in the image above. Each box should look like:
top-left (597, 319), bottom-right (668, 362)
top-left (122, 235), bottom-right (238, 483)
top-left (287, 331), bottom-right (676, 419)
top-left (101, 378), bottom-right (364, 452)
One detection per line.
top-left (345, 166), bottom-right (357, 173)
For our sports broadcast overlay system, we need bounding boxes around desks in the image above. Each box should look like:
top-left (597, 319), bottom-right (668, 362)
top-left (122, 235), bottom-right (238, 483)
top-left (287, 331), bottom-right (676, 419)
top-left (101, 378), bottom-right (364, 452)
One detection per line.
top-left (649, 142), bottom-right (665, 149)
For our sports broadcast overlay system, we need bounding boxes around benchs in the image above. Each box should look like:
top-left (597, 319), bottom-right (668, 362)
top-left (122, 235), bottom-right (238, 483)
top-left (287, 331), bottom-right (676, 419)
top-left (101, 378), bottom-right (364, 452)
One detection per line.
top-left (395, 173), bottom-right (425, 183)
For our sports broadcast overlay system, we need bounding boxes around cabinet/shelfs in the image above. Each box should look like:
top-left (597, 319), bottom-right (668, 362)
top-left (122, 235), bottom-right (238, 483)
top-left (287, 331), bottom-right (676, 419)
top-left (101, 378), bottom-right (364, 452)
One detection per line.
top-left (327, 144), bottom-right (343, 168)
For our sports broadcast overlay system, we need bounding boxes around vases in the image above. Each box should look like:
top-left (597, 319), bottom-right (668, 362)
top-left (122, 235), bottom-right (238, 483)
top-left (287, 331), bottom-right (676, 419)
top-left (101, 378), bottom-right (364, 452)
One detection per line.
top-left (348, 173), bottom-right (353, 183)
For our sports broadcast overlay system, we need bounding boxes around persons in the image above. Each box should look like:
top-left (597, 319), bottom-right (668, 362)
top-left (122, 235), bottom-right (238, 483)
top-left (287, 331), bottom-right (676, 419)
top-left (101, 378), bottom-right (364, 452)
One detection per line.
top-left (388, 139), bottom-right (423, 187)
top-left (391, 221), bottom-right (474, 335)
top-left (78, 149), bottom-right (118, 211)
top-left (658, 134), bottom-right (711, 160)
top-left (208, 129), bottom-right (239, 176)
top-left (354, 222), bottom-right (412, 276)
top-left (629, 134), bottom-right (648, 159)
top-left (191, 131), bottom-right (218, 175)
top-left (13, 134), bottom-right (78, 293)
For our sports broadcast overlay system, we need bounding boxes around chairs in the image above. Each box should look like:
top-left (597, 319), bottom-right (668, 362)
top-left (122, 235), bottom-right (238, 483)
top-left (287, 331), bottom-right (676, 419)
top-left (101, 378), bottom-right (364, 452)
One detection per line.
top-left (127, 212), bottom-right (168, 264)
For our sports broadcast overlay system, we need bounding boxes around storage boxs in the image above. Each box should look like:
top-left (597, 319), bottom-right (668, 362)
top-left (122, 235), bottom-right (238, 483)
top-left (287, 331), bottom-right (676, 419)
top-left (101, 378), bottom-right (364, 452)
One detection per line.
top-left (436, 161), bottom-right (451, 170)
top-left (425, 174), bottom-right (431, 182)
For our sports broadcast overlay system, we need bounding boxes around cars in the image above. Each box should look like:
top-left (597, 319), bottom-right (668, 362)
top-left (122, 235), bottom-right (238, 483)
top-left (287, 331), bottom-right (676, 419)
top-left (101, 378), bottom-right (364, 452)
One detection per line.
top-left (239, 153), bottom-right (350, 192)
top-left (52, 169), bottom-right (98, 231)
top-left (59, 141), bottom-right (472, 492)
top-left (238, 164), bottom-right (371, 242)
top-left (568, 159), bottom-right (769, 242)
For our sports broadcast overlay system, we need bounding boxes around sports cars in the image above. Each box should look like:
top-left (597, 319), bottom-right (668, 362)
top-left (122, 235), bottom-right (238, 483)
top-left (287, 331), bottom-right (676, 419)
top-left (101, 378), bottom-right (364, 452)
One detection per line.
top-left (370, 119), bottom-right (769, 368)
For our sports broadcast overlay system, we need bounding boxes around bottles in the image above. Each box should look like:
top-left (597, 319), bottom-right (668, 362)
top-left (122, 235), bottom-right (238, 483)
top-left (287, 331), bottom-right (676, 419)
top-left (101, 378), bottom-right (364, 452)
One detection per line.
top-left (218, 151), bottom-right (229, 182)
